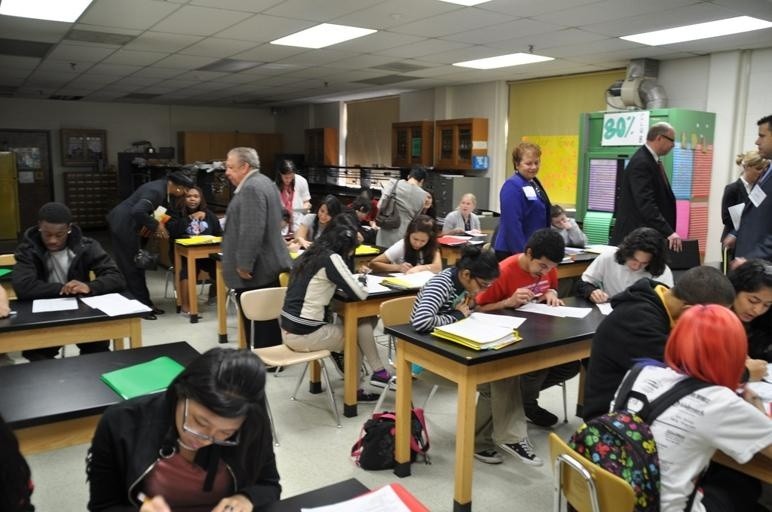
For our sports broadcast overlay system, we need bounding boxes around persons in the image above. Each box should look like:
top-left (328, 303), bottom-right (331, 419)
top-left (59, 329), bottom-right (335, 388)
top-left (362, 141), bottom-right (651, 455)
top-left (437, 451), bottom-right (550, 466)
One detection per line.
top-left (721, 116), bottom-right (771, 267)
top-left (610, 121), bottom-right (682, 252)
top-left (221, 148), bottom-right (284, 373)
top-left (582, 261), bottom-right (771, 512)
top-left (281, 167), bottom-right (478, 403)
top-left (552, 205), bottom-right (585, 247)
top-left (87, 346), bottom-right (282, 512)
top-left (106, 171), bottom-right (193, 319)
top-left (11, 200), bottom-right (120, 362)
top-left (164, 185), bottom-right (222, 306)
top-left (273, 157), bottom-right (312, 214)
top-left (406, 229), bottom-right (581, 466)
top-left (580, 227), bottom-right (674, 303)
top-left (494, 143), bottom-right (552, 261)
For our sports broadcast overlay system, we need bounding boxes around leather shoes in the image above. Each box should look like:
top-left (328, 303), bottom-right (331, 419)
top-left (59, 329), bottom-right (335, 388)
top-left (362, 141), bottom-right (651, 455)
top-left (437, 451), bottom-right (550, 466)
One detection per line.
top-left (143, 304), bottom-right (164, 320)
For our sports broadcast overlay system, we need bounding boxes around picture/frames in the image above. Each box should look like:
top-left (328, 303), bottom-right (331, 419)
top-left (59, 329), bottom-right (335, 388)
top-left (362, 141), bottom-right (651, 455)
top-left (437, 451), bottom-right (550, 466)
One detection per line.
top-left (60, 127), bottom-right (108, 166)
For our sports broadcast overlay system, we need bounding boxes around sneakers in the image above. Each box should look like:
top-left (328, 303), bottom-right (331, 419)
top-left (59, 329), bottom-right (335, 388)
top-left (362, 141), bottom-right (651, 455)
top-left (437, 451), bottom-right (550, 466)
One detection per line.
top-left (370, 373), bottom-right (397, 391)
top-left (474, 448), bottom-right (503, 464)
top-left (208, 296), bottom-right (217, 306)
top-left (495, 436), bottom-right (542, 466)
top-left (526, 401), bottom-right (558, 427)
top-left (331, 350), bottom-right (345, 377)
top-left (357, 388), bottom-right (381, 403)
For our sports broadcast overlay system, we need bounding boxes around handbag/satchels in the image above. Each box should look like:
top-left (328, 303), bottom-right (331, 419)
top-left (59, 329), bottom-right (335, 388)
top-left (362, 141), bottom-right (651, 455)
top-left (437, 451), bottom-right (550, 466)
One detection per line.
top-left (352, 408), bottom-right (429, 471)
top-left (134, 246), bottom-right (162, 271)
top-left (375, 197), bottom-right (401, 229)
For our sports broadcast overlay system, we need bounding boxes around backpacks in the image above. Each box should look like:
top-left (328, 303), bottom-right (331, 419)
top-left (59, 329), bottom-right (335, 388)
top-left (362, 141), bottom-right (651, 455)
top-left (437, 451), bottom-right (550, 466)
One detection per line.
top-left (564, 367), bottom-right (696, 512)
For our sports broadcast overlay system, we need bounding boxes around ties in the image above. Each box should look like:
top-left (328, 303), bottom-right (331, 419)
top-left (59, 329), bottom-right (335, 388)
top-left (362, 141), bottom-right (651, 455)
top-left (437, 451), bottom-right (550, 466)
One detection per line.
top-left (658, 158), bottom-right (670, 192)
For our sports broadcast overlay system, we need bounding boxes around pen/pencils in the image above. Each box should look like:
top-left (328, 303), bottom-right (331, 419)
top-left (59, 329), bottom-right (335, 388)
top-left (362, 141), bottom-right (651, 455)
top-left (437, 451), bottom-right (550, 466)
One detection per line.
top-left (532, 277), bottom-right (542, 293)
top-left (136, 491), bottom-right (152, 503)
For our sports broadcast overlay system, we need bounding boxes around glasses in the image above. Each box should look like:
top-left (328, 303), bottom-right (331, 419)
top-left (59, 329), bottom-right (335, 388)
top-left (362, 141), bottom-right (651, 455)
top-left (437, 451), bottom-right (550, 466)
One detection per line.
top-left (661, 134), bottom-right (675, 142)
top-left (181, 397), bottom-right (243, 448)
top-left (475, 278), bottom-right (493, 289)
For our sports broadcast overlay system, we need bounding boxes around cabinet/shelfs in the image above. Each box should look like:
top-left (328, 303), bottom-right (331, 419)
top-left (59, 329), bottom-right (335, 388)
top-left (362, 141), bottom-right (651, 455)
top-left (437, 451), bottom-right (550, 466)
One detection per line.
top-left (65, 169), bottom-right (118, 230)
top-left (117, 151), bottom-right (175, 201)
top-left (178, 130), bottom-right (284, 204)
top-left (1, 150), bottom-right (21, 240)
top-left (305, 127), bottom-right (338, 167)
top-left (423, 175), bottom-right (489, 216)
top-left (577, 108), bottom-right (715, 267)
top-left (391, 121), bottom-right (431, 166)
top-left (432, 117), bottom-right (490, 172)
